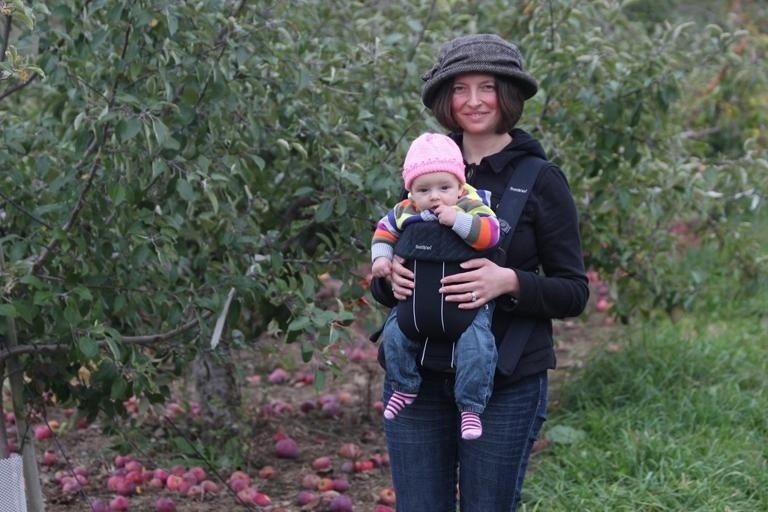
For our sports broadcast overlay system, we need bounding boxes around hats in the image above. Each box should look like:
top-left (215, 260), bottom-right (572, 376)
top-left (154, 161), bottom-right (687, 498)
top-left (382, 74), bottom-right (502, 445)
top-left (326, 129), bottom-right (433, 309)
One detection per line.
top-left (402, 132), bottom-right (466, 190)
top-left (420, 33), bottom-right (537, 107)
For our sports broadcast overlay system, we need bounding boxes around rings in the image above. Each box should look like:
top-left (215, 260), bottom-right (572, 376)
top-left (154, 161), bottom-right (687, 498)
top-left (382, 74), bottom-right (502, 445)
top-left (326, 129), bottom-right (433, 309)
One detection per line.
top-left (471, 291), bottom-right (476, 302)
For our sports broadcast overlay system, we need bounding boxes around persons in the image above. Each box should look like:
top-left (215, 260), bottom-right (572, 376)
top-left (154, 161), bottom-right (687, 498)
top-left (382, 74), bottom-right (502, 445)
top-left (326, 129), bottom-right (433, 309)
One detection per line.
top-left (371, 34), bottom-right (590, 511)
top-left (371, 133), bottom-right (501, 440)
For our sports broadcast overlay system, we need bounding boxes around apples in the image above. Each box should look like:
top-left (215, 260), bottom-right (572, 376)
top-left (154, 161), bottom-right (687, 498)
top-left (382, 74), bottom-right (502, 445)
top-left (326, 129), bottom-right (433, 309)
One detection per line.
top-left (2, 274), bottom-right (397, 512)
top-left (586, 271), bottom-right (615, 325)
